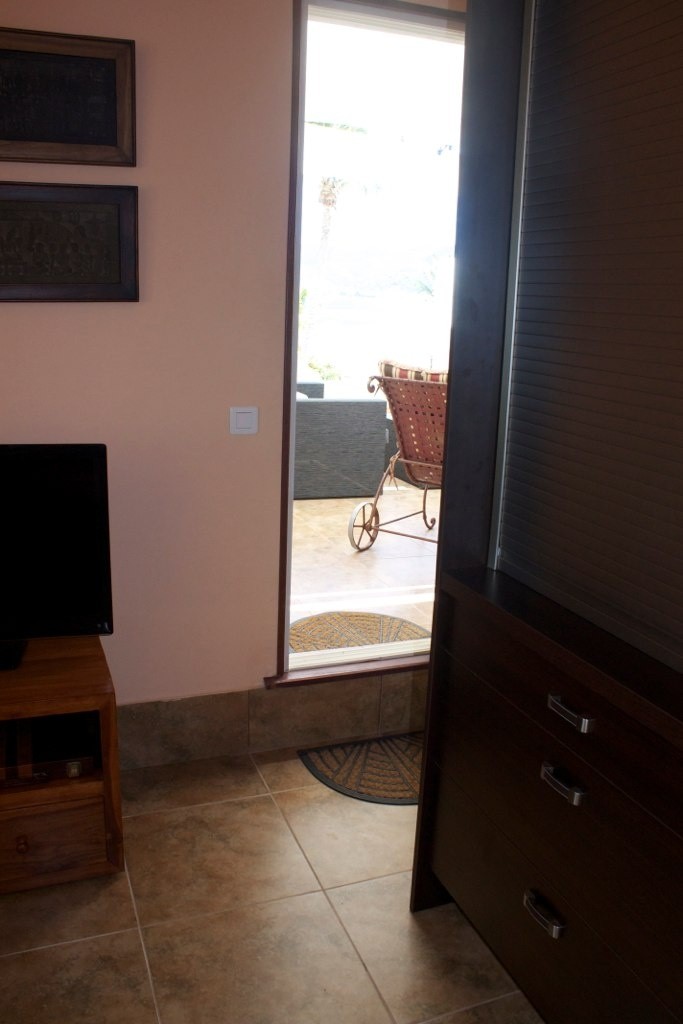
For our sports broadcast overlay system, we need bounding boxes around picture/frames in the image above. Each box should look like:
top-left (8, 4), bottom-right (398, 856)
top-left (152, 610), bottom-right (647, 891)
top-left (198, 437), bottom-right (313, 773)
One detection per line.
top-left (0, 182), bottom-right (139, 303)
top-left (0, 27), bottom-right (137, 167)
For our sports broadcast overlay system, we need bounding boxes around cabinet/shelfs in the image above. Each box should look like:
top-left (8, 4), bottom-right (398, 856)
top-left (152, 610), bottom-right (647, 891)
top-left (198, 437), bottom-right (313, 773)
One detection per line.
top-left (0, 627), bottom-right (126, 893)
top-left (409, 1), bottom-right (683, 1024)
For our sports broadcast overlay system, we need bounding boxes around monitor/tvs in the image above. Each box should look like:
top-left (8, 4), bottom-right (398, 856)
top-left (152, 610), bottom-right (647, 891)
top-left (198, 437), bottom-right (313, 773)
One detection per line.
top-left (0, 443), bottom-right (114, 671)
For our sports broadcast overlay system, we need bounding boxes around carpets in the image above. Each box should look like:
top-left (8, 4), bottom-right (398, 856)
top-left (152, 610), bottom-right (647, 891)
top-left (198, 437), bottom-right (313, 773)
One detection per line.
top-left (295, 730), bottom-right (426, 805)
top-left (288, 613), bottom-right (431, 655)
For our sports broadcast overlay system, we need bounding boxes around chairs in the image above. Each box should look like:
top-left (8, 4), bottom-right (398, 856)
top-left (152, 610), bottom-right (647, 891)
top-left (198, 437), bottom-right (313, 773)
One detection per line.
top-left (346, 364), bottom-right (452, 552)
top-left (295, 383), bottom-right (389, 496)
